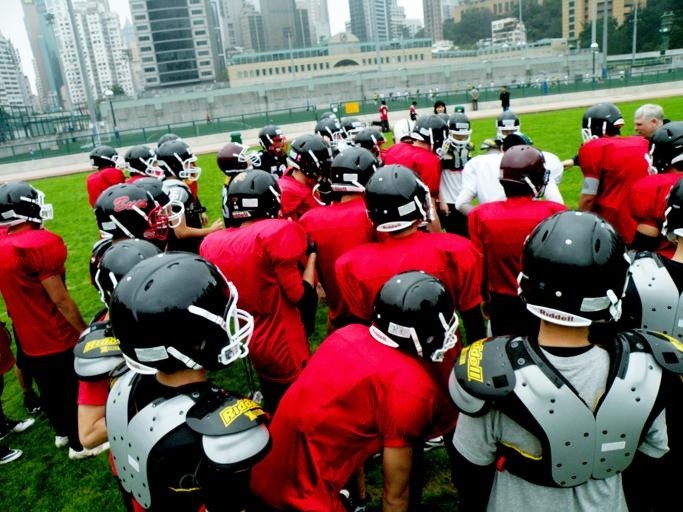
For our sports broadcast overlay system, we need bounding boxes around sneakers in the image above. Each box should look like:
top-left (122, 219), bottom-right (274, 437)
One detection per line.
top-left (68, 441), bottom-right (110, 460)
top-left (54, 435), bottom-right (70, 449)
top-left (1, 417), bottom-right (36, 439)
top-left (0, 446), bottom-right (23, 464)
top-left (23, 394), bottom-right (41, 414)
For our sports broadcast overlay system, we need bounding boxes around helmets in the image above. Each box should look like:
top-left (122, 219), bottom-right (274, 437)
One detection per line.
top-left (0, 182), bottom-right (53, 231)
top-left (218, 110), bottom-right (472, 237)
top-left (496, 111), bottom-right (521, 139)
top-left (581, 104), bottom-right (625, 143)
top-left (518, 211), bottom-right (630, 329)
top-left (372, 271), bottom-right (459, 365)
top-left (499, 144), bottom-right (549, 198)
top-left (645, 121), bottom-right (683, 174)
top-left (89, 133), bottom-right (253, 376)
top-left (661, 176), bottom-right (683, 239)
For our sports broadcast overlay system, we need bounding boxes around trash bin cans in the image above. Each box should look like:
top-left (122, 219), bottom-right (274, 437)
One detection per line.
top-left (231, 132), bottom-right (241, 144)
top-left (455, 106), bottom-right (464, 112)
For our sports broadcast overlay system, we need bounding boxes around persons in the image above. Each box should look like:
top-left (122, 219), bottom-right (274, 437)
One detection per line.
top-left (470, 86), bottom-right (479, 111)
top-left (0, 321), bottom-right (35, 464)
top-left (64, 101), bottom-right (682, 512)
top-left (13, 305), bottom-right (39, 415)
top-left (0, 181), bottom-right (102, 458)
top-left (498, 85), bottom-right (510, 112)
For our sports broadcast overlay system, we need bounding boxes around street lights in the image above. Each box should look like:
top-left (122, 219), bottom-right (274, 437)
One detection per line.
top-left (42, 10), bottom-right (76, 121)
top-left (588, 43), bottom-right (600, 90)
top-left (105, 90), bottom-right (123, 147)
top-left (659, 11), bottom-right (675, 58)
top-left (283, 24), bottom-right (298, 80)
